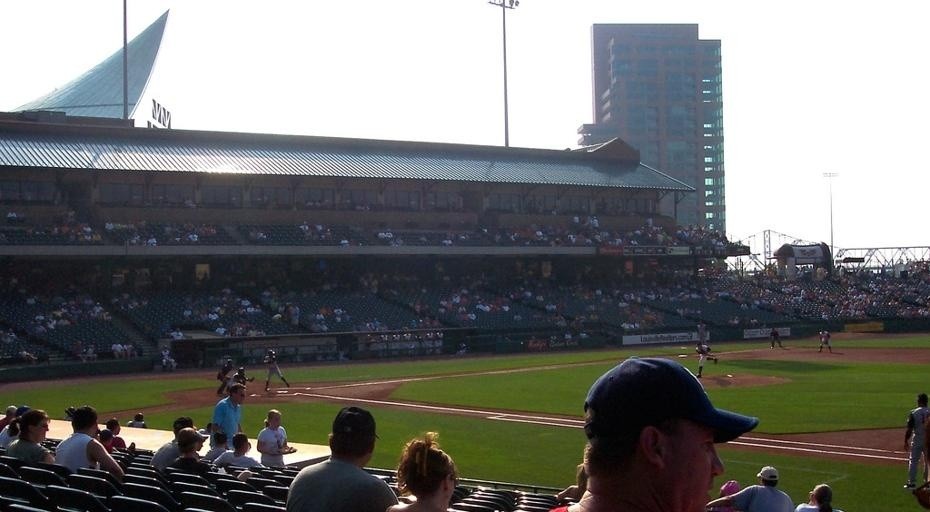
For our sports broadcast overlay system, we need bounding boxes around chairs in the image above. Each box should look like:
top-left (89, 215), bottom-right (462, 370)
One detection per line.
top-left (0, 432), bottom-right (575, 511)
top-left (2, 198), bottom-right (929, 372)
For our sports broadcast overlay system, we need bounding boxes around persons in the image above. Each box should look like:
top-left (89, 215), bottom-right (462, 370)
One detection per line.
top-left (547, 356), bottom-right (759, 512)
top-left (557, 464), bottom-right (588, 503)
top-left (210, 349), bottom-right (289, 447)
top-left (707, 466), bottom-right (836, 512)
top-left (0, 405), bottom-right (460, 512)
top-left (695, 342), bottom-right (718, 378)
top-left (903, 394), bottom-right (930, 489)
top-left (2, 212), bottom-right (930, 370)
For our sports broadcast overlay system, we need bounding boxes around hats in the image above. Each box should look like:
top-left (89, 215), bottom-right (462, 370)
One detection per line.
top-left (583, 356), bottom-right (760, 443)
top-left (196, 430), bottom-right (208, 440)
top-left (720, 479), bottom-right (738, 495)
top-left (332, 408), bottom-right (383, 438)
top-left (757, 465), bottom-right (779, 481)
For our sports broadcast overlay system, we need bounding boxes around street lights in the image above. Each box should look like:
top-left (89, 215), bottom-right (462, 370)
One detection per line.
top-left (488, 0), bottom-right (520, 151)
top-left (824, 172), bottom-right (839, 279)
